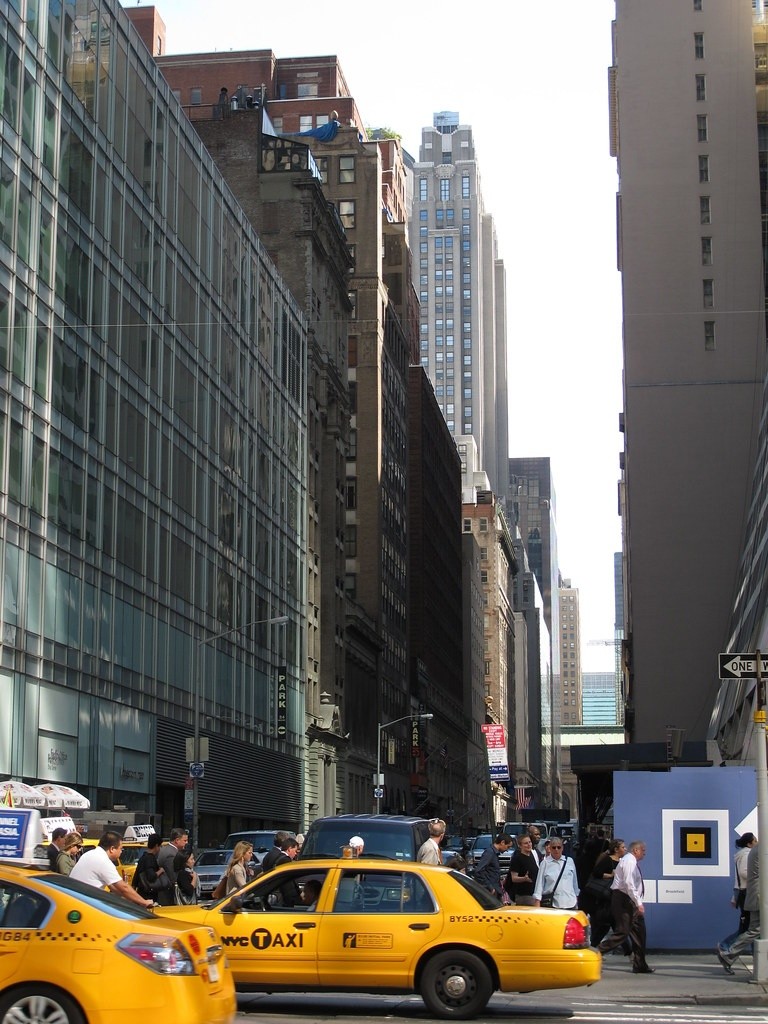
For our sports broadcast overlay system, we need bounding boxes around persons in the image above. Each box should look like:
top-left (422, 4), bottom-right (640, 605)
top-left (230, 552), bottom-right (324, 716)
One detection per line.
top-left (298, 880), bottom-right (323, 912)
top-left (47, 827), bottom-right (203, 909)
top-left (225, 832), bottom-right (303, 908)
top-left (349, 836), bottom-right (364, 857)
top-left (475, 824), bottom-right (654, 974)
top-left (417, 818), bottom-right (446, 866)
top-left (716, 832), bottom-right (759, 975)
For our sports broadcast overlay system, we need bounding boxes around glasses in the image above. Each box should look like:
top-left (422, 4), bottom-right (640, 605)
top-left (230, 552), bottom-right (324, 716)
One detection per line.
top-left (116, 847), bottom-right (125, 851)
top-left (75, 844), bottom-right (81, 849)
top-left (553, 846), bottom-right (561, 849)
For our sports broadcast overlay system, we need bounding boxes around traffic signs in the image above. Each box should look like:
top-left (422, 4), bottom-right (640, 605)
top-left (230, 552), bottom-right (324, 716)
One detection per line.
top-left (718, 653), bottom-right (768, 679)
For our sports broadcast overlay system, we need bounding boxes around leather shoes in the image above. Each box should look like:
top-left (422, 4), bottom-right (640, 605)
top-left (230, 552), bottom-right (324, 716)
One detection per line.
top-left (718, 953), bottom-right (735, 975)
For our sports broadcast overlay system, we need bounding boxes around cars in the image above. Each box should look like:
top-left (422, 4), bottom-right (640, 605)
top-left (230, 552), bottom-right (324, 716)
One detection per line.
top-left (194, 847), bottom-right (263, 899)
top-left (440, 835), bottom-right (475, 853)
top-left (40, 817), bottom-right (171, 893)
top-left (438, 851), bottom-right (466, 869)
top-left (0, 807), bottom-right (238, 1023)
top-left (148, 857), bottom-right (603, 1020)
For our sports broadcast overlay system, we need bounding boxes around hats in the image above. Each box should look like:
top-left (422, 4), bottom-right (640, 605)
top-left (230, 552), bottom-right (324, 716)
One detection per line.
top-left (349, 836), bottom-right (365, 847)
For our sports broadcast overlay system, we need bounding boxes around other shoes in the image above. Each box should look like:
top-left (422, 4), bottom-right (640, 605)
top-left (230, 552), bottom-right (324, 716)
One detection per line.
top-left (629, 955), bottom-right (634, 961)
top-left (634, 968), bottom-right (655, 973)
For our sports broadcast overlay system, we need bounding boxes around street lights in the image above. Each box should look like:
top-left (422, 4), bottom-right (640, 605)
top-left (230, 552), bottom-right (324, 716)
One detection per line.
top-left (193, 616), bottom-right (291, 843)
top-left (377, 713), bottom-right (434, 813)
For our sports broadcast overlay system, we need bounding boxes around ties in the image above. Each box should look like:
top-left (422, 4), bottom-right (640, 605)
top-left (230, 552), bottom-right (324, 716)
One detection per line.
top-left (636, 864), bottom-right (644, 896)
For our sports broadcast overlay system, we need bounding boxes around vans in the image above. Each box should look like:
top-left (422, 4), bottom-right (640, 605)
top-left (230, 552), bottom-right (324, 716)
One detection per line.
top-left (221, 830), bottom-right (298, 863)
top-left (502, 823), bottom-right (559, 856)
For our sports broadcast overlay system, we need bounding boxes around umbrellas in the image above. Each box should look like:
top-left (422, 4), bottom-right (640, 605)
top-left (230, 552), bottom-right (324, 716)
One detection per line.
top-left (0, 780), bottom-right (90, 818)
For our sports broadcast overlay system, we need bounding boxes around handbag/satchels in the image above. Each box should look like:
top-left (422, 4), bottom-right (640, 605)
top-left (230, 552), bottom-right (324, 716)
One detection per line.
top-left (586, 878), bottom-right (613, 898)
top-left (140, 870), bottom-right (172, 891)
top-left (736, 889), bottom-right (746, 908)
top-left (540, 892), bottom-right (554, 907)
top-left (174, 884), bottom-right (197, 905)
top-left (212, 876), bottom-right (226, 898)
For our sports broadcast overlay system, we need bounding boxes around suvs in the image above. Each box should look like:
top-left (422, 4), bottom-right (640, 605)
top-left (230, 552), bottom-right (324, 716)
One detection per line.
top-left (467, 836), bottom-right (517, 871)
top-left (298, 814), bottom-right (446, 910)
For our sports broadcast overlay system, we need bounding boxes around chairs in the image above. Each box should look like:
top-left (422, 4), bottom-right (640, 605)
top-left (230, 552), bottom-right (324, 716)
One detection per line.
top-left (4, 895), bottom-right (38, 927)
